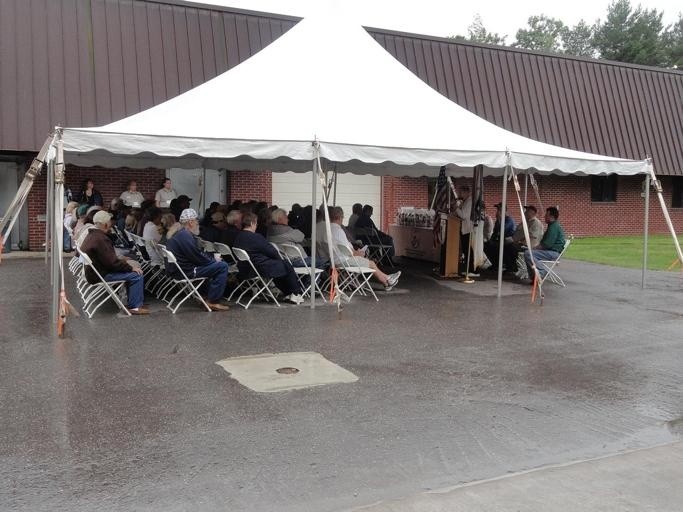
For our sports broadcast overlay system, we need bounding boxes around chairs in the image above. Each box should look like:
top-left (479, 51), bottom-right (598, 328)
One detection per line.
top-left (534, 237), bottom-right (570, 288)
top-left (515, 244), bottom-right (528, 280)
top-left (63, 220), bottom-right (397, 320)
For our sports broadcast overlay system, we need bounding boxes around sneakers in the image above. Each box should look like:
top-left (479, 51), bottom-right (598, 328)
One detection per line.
top-left (289, 292), bottom-right (305, 303)
top-left (383, 279), bottom-right (398, 292)
top-left (202, 298), bottom-right (230, 312)
top-left (388, 270), bottom-right (402, 286)
top-left (124, 305), bottom-right (152, 315)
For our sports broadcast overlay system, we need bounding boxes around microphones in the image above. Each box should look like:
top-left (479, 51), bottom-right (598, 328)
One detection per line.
top-left (455, 197), bottom-right (463, 200)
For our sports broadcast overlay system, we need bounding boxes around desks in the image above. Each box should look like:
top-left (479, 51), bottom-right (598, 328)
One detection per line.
top-left (387, 222), bottom-right (441, 264)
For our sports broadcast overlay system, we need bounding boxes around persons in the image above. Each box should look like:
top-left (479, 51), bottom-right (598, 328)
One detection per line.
top-left (64, 178), bottom-right (401, 315)
top-left (455, 186), bottom-right (565, 286)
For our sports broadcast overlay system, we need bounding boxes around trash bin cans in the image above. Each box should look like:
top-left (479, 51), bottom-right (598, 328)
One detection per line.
top-left (1, 219), bottom-right (11, 253)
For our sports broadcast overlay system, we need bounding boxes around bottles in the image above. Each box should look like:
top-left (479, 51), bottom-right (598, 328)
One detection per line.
top-left (395, 207), bottom-right (434, 227)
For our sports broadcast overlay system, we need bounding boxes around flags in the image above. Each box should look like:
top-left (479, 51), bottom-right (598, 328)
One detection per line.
top-left (432, 164), bottom-right (457, 248)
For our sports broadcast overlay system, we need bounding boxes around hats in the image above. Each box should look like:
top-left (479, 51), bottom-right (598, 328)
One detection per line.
top-left (177, 194), bottom-right (192, 202)
top-left (92, 209), bottom-right (114, 226)
top-left (179, 208), bottom-right (199, 221)
top-left (66, 199), bottom-right (102, 217)
top-left (493, 201), bottom-right (508, 212)
top-left (523, 205), bottom-right (537, 214)
